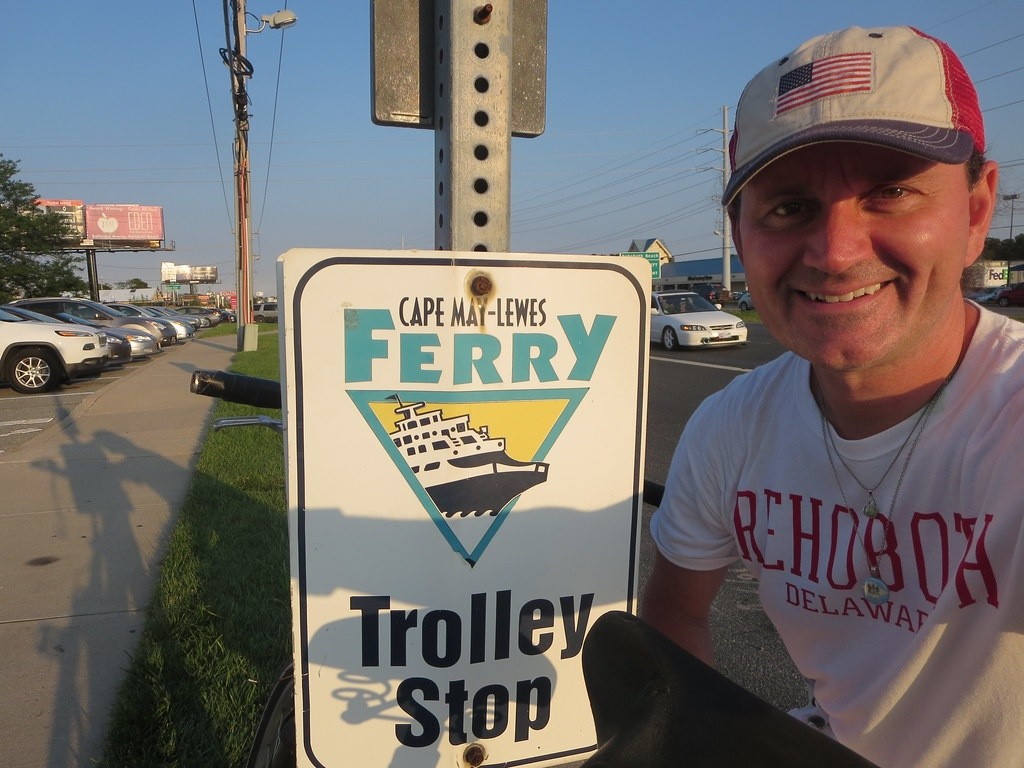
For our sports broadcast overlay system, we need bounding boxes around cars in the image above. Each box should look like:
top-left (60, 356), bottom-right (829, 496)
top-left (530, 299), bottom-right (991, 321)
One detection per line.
top-left (110, 326), bottom-right (162, 357)
top-left (130, 317), bottom-right (177, 347)
top-left (651, 289), bottom-right (747, 350)
top-left (77, 304), bottom-right (196, 339)
top-left (689, 284), bottom-right (729, 308)
top-left (52, 313), bottom-right (132, 367)
top-left (0, 307), bottom-right (109, 395)
top-left (120, 306), bottom-right (231, 331)
top-left (253, 302), bottom-right (278, 323)
top-left (4, 296), bottom-right (163, 343)
top-left (996, 282), bottom-right (1024, 307)
top-left (739, 291), bottom-right (753, 310)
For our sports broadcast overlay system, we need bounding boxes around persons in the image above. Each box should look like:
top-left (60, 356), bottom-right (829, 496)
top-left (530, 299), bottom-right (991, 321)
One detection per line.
top-left (640, 25), bottom-right (1024, 768)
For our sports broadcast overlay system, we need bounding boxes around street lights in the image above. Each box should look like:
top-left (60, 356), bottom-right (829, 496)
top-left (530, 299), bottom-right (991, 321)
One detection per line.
top-left (714, 231), bottom-right (732, 291)
top-left (227, 10), bottom-right (299, 351)
top-left (1003, 194), bottom-right (1020, 288)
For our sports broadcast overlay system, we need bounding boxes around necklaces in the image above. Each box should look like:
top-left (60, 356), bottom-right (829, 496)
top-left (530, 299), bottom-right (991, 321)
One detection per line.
top-left (812, 368), bottom-right (955, 605)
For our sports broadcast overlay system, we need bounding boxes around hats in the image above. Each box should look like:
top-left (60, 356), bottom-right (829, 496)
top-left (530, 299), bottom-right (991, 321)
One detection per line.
top-left (721, 26), bottom-right (987, 209)
